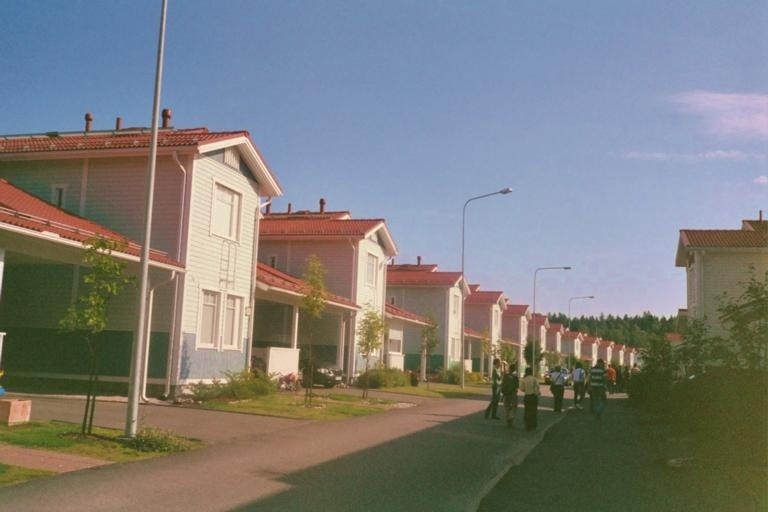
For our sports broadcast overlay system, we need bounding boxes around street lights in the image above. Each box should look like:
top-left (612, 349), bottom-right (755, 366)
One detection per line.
top-left (531, 266), bottom-right (572, 375)
top-left (460, 186), bottom-right (513, 389)
top-left (568, 295), bottom-right (595, 368)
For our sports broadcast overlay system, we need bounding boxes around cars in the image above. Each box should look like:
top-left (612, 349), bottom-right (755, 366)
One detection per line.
top-left (544, 366), bottom-right (573, 386)
top-left (253, 341), bottom-right (344, 388)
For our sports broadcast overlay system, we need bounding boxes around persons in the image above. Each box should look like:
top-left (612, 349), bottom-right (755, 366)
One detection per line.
top-left (518, 366), bottom-right (541, 432)
top-left (548, 365), bottom-right (565, 414)
top-left (484, 359), bottom-right (502, 420)
top-left (500, 363), bottom-right (519, 430)
top-left (586, 357), bottom-right (609, 422)
top-left (604, 363), bottom-right (642, 395)
top-left (570, 361), bottom-right (585, 408)
